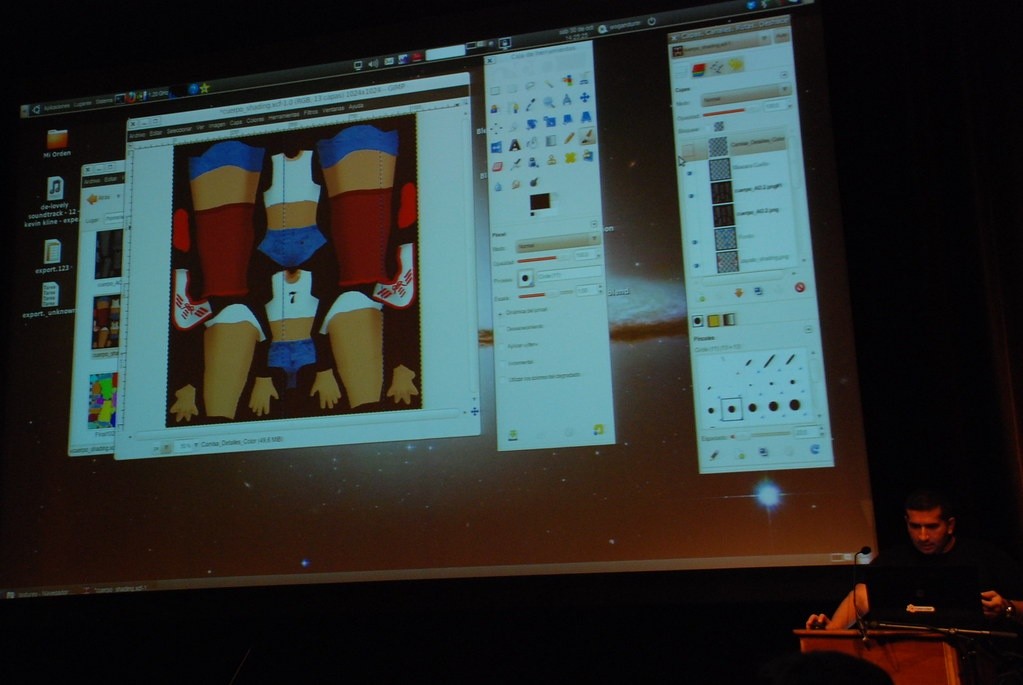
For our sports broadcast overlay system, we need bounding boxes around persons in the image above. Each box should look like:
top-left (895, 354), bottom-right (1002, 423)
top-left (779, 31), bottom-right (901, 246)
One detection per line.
top-left (759, 651), bottom-right (894, 685)
top-left (805, 486), bottom-right (1023, 633)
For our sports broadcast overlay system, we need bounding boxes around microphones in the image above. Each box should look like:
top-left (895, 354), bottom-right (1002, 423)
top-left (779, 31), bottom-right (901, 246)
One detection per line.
top-left (853, 546), bottom-right (872, 640)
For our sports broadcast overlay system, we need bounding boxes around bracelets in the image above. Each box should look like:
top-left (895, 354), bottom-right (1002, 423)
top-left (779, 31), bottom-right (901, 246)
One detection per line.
top-left (1005, 601), bottom-right (1016, 619)
